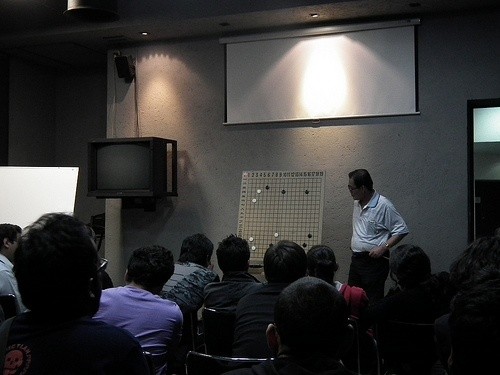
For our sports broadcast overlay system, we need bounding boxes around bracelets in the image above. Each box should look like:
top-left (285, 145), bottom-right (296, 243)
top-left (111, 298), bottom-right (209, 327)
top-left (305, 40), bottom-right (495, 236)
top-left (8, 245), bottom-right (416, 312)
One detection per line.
top-left (383, 242), bottom-right (391, 248)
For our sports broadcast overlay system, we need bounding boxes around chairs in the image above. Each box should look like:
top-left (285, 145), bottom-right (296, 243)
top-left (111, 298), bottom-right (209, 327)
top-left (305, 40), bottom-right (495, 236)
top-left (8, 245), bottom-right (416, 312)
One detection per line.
top-left (0, 292), bottom-right (500, 374)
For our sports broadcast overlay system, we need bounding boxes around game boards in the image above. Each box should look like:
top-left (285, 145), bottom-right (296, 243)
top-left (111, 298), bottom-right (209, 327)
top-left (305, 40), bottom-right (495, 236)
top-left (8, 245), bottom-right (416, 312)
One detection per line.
top-left (236, 169), bottom-right (326, 266)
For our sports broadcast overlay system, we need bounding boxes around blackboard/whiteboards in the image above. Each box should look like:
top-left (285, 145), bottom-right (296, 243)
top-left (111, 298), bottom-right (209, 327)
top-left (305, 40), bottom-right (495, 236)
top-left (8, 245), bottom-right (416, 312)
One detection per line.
top-left (0, 167), bottom-right (80, 237)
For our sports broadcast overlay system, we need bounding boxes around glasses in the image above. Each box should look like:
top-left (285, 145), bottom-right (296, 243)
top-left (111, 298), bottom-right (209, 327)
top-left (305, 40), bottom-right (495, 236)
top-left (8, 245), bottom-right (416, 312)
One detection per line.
top-left (350, 186), bottom-right (362, 192)
top-left (92, 258), bottom-right (108, 278)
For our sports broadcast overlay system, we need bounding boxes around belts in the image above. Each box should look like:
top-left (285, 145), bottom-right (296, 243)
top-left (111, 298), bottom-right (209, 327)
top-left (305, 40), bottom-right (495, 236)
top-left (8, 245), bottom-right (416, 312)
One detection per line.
top-left (353, 252), bottom-right (370, 255)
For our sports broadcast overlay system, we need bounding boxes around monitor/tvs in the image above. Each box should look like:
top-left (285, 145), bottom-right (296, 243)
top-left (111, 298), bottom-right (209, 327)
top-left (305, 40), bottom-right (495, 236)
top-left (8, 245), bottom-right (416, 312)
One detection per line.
top-left (90, 141), bottom-right (167, 193)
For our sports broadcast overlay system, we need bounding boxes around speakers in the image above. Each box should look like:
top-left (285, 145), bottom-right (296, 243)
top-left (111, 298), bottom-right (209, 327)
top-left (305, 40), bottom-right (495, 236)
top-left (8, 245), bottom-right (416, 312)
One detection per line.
top-left (115, 54), bottom-right (136, 78)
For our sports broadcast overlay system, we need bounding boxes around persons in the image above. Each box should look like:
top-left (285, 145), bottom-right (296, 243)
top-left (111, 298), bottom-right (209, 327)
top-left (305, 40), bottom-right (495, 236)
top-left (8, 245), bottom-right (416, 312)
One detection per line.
top-left (91, 245), bottom-right (184, 374)
top-left (0, 213), bottom-right (146, 374)
top-left (307, 245), bottom-right (368, 318)
top-left (202, 235), bottom-right (263, 308)
top-left (434, 231), bottom-right (499, 374)
top-left (237, 241), bottom-right (308, 358)
top-left (1, 223), bottom-right (26, 316)
top-left (233, 277), bottom-right (357, 374)
top-left (151, 231), bottom-right (220, 307)
top-left (368, 245), bottom-right (453, 328)
top-left (346, 168), bottom-right (408, 298)
